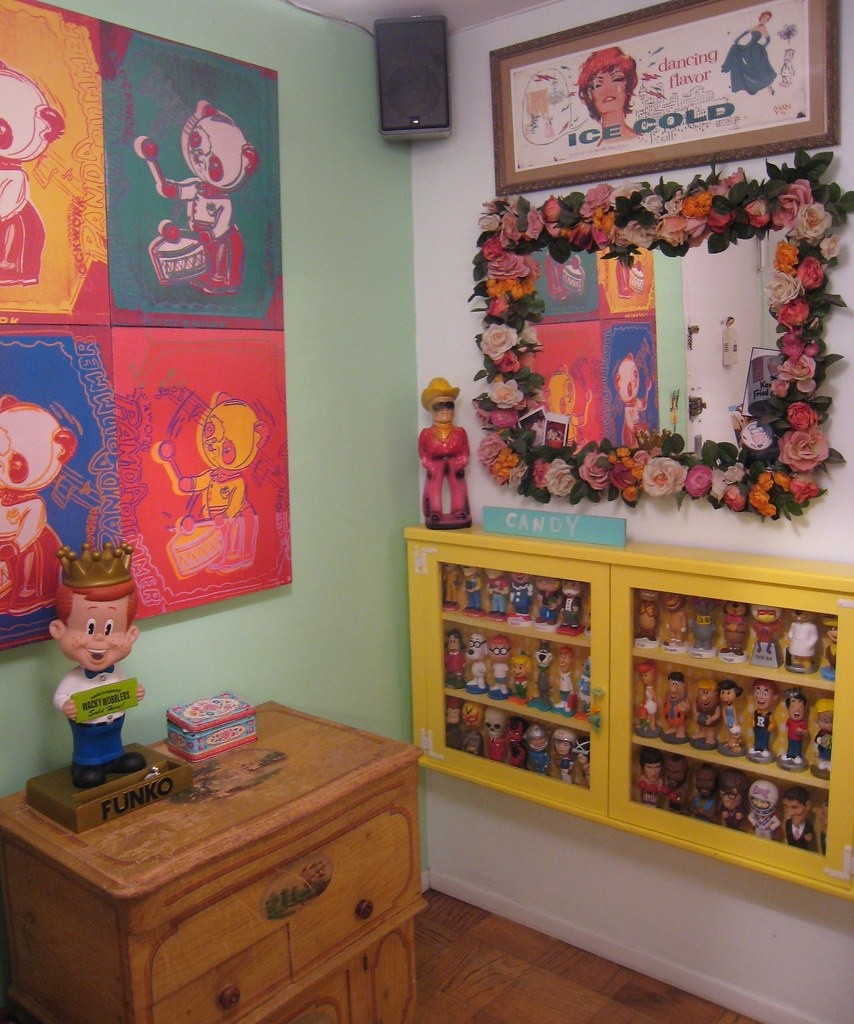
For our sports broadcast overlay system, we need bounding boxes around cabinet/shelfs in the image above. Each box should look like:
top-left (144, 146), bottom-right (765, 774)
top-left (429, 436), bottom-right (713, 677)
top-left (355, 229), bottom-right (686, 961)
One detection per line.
top-left (403, 521), bottom-right (854, 903)
top-left (0, 700), bottom-right (425, 1024)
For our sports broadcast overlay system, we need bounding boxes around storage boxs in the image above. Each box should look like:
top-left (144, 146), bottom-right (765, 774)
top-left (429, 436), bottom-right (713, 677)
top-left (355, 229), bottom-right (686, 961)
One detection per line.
top-left (166, 690), bottom-right (258, 763)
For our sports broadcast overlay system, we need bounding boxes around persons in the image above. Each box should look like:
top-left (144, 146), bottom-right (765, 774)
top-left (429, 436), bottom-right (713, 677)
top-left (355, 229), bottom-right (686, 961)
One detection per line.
top-left (442, 629), bottom-right (592, 720)
top-left (419, 377), bottom-right (470, 524)
top-left (443, 696), bottom-right (591, 788)
top-left (50, 542), bottom-right (145, 788)
top-left (633, 586), bottom-right (839, 674)
top-left (635, 746), bottom-right (828, 856)
top-left (440, 562), bottom-right (591, 636)
top-left (635, 661), bottom-right (834, 773)
top-left (531, 417), bottom-right (545, 445)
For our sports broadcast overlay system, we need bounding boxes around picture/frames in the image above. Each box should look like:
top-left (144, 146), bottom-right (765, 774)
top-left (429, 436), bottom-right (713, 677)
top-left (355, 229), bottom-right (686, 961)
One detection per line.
top-left (489, 1), bottom-right (842, 197)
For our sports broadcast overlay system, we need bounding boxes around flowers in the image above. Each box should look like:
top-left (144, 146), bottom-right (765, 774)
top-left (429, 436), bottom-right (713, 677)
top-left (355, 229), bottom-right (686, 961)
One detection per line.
top-left (466, 149), bottom-right (854, 523)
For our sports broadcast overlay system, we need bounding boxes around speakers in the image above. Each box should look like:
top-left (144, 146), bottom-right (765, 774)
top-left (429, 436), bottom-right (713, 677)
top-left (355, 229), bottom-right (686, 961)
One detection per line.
top-left (372, 14), bottom-right (450, 135)
